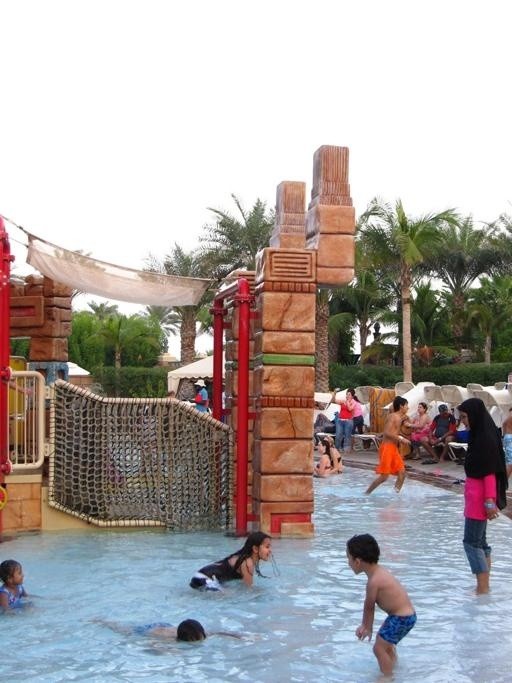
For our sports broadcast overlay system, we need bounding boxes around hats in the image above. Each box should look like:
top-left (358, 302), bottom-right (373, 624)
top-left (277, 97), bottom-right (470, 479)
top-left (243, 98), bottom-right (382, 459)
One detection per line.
top-left (195, 379), bottom-right (206, 388)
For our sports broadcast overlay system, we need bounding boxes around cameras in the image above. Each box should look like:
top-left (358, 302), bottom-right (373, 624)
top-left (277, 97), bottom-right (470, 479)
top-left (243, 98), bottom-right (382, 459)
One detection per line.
top-left (334, 387), bottom-right (341, 391)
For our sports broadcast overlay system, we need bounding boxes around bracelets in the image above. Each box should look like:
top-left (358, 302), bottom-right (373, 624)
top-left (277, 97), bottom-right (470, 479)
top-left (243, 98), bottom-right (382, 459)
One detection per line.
top-left (484, 501), bottom-right (496, 508)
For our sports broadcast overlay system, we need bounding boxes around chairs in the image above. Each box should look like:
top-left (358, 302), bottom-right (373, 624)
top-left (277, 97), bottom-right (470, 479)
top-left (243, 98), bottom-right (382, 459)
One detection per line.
top-left (313, 375), bottom-right (512, 470)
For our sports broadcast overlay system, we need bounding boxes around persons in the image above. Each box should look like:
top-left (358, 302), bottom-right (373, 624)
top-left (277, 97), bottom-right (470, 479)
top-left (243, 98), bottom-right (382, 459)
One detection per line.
top-left (166, 390), bottom-right (175, 398)
top-left (221, 382), bottom-right (226, 424)
top-left (138, 406), bottom-right (158, 465)
top-left (345, 533), bottom-right (417, 682)
top-left (189, 378), bottom-right (210, 413)
top-left (313, 386), bottom-right (456, 495)
top-left (79, 618), bottom-right (262, 655)
top-left (189, 530), bottom-right (273, 598)
top-left (501, 406), bottom-right (512, 479)
top-left (456, 396), bottom-right (509, 593)
top-left (0, 559), bottom-right (43, 616)
